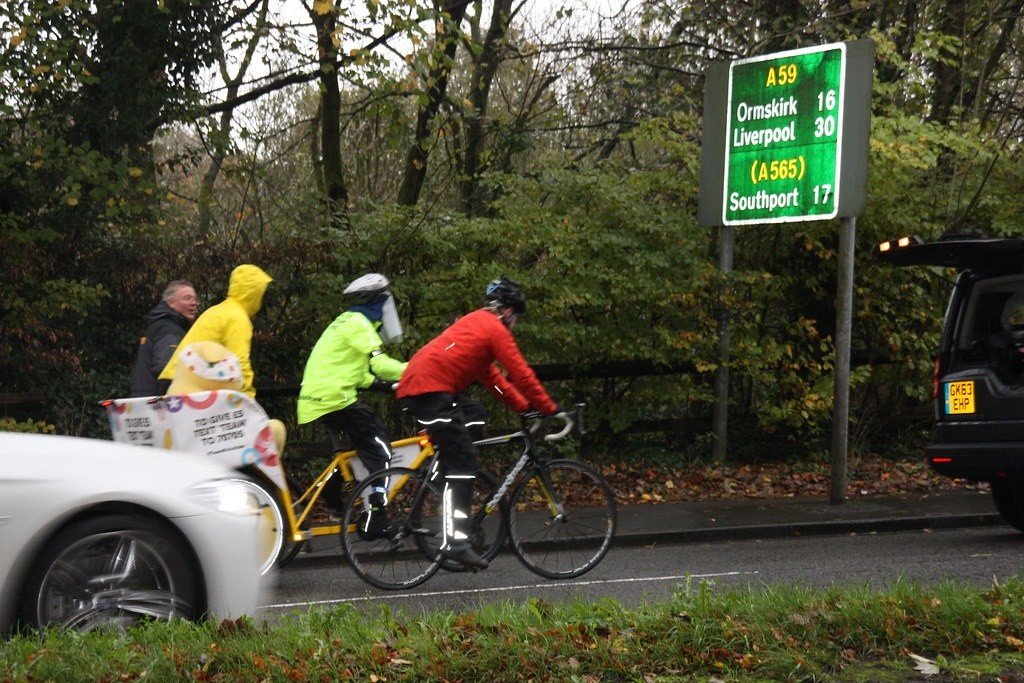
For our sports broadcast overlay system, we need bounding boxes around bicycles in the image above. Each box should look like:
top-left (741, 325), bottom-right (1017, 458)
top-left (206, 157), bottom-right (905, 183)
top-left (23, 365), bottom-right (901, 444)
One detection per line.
top-left (339, 403), bottom-right (617, 591)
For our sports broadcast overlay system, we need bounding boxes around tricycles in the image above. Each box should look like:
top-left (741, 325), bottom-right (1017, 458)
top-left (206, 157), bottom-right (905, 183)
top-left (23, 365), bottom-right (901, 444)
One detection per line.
top-left (97, 378), bottom-right (510, 573)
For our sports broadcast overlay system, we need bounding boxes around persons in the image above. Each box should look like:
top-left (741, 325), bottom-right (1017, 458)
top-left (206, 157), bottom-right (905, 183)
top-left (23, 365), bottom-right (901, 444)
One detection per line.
top-left (158, 265), bottom-right (274, 400)
top-left (397, 278), bottom-right (570, 571)
top-left (129, 280), bottom-right (197, 398)
top-left (297, 274), bottom-right (411, 548)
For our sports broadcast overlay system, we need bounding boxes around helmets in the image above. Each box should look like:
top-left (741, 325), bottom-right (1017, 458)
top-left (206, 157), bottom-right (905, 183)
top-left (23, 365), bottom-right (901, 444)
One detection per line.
top-left (344, 272), bottom-right (391, 304)
top-left (488, 277), bottom-right (527, 314)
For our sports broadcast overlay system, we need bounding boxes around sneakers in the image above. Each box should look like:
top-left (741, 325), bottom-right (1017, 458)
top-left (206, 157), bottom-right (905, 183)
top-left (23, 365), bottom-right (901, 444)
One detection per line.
top-left (358, 507), bottom-right (412, 540)
top-left (319, 479), bottom-right (344, 508)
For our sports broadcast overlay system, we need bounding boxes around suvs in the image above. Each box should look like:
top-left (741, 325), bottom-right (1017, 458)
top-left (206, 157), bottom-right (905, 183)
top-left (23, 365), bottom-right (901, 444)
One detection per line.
top-left (874, 233), bottom-right (1024, 534)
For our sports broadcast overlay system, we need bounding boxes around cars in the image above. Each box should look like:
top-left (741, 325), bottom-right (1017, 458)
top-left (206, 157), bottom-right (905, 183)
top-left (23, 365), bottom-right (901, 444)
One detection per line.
top-left (0, 430), bottom-right (265, 642)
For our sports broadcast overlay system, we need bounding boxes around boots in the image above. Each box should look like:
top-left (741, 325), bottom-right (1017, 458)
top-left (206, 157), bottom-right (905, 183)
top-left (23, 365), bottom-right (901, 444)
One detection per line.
top-left (436, 471), bottom-right (489, 569)
